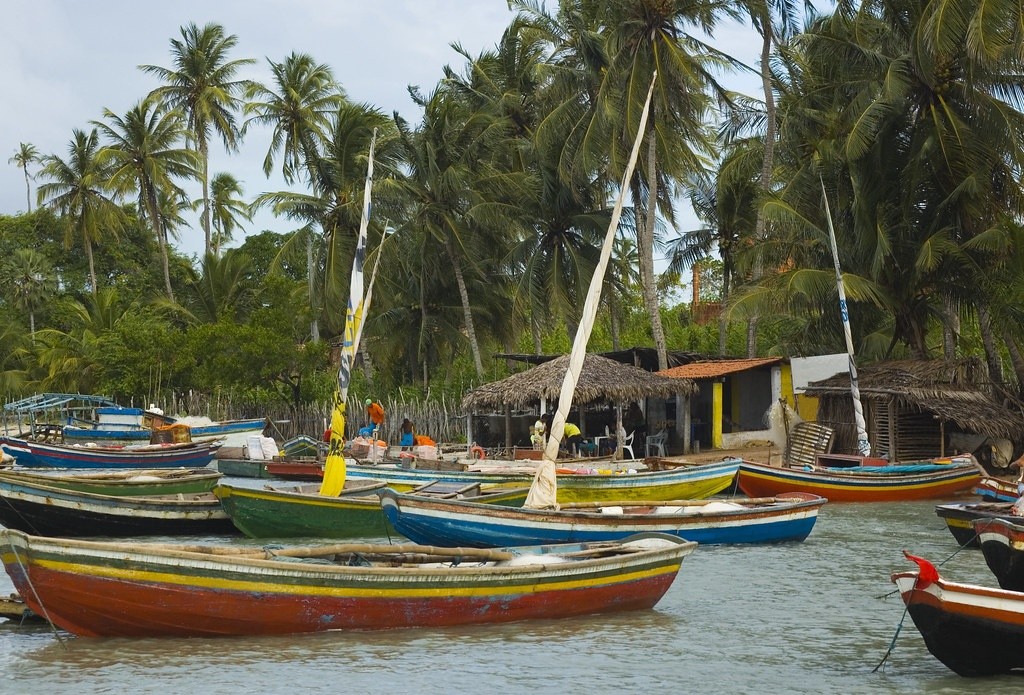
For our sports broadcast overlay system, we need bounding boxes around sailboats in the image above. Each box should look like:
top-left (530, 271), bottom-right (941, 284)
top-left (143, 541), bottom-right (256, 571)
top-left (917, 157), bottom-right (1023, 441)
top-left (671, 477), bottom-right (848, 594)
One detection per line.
top-left (722, 171), bottom-right (984, 501)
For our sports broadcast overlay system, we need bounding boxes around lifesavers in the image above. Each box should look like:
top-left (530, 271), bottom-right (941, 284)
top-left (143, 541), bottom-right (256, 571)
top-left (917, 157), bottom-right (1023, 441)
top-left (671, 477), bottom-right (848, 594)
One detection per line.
top-left (469, 446), bottom-right (486, 461)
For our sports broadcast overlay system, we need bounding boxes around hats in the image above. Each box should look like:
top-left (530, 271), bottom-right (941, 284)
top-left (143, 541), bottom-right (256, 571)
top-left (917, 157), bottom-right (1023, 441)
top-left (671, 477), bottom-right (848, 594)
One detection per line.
top-left (365, 398), bottom-right (372, 405)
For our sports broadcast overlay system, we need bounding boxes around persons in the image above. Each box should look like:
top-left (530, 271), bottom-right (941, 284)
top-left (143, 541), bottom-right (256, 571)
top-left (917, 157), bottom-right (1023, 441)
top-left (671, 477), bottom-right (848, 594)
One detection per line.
top-left (366, 398), bottom-right (386, 439)
top-left (400, 418), bottom-right (416, 451)
top-left (1009, 453), bottom-right (1024, 515)
top-left (535, 414), bottom-right (626, 458)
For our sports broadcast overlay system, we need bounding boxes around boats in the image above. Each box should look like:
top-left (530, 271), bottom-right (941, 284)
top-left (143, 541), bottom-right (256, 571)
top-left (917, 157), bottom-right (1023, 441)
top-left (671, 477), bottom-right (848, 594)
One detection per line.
top-left (890, 548), bottom-right (1024, 676)
top-left (0, 528), bottom-right (700, 638)
top-left (374, 485), bottom-right (827, 549)
top-left (933, 475), bottom-right (1024, 592)
top-left (0, 70), bottom-right (742, 541)
